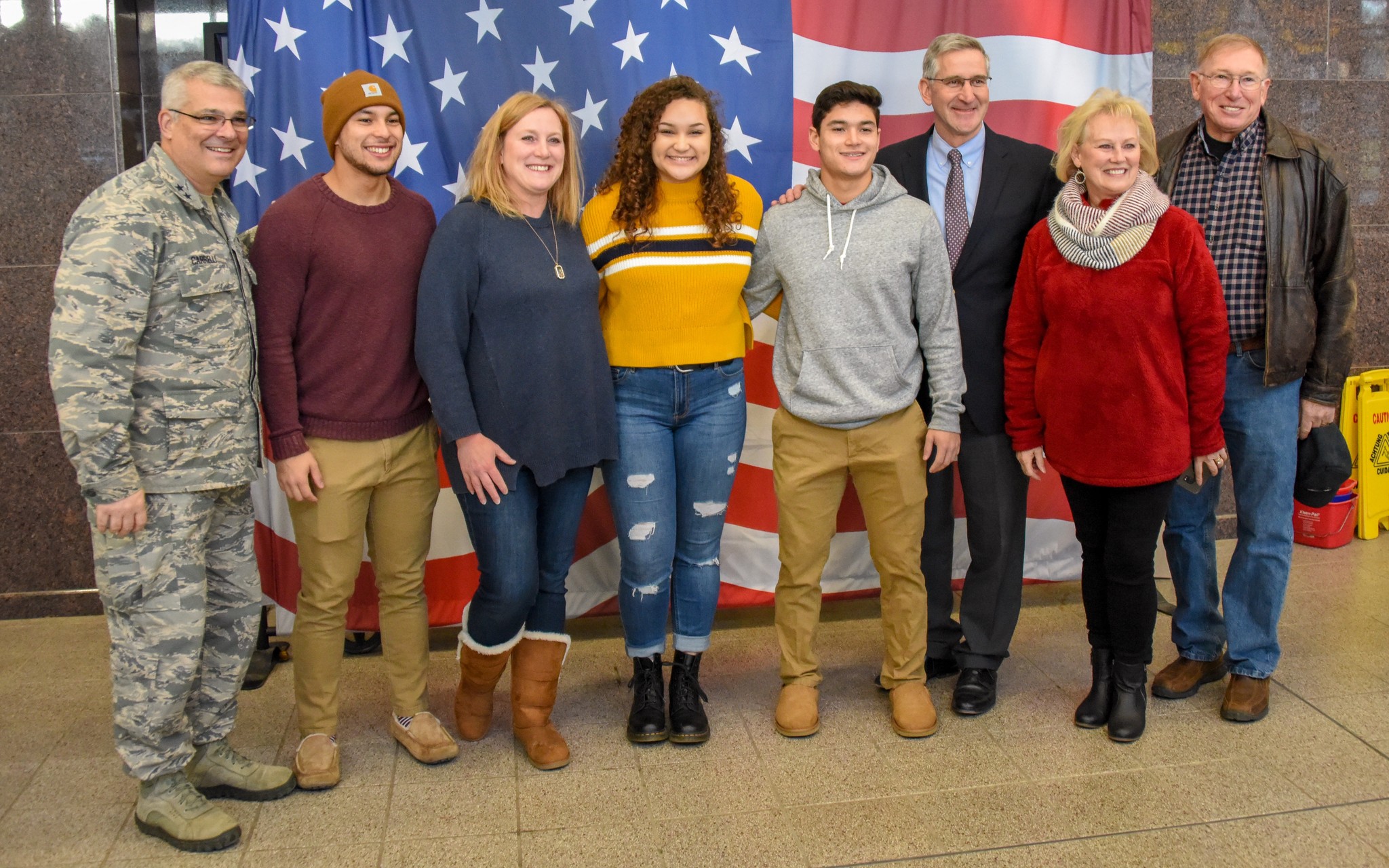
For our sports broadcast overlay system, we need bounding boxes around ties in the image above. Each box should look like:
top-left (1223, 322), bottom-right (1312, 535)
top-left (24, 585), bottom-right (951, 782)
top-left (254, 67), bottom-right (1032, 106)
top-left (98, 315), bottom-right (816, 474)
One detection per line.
top-left (945, 149), bottom-right (970, 277)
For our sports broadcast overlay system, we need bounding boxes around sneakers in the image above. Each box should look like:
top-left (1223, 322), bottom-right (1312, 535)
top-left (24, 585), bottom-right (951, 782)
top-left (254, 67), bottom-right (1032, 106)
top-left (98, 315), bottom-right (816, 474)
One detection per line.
top-left (184, 737), bottom-right (296, 801)
top-left (1221, 673), bottom-right (1270, 722)
top-left (393, 711), bottom-right (458, 765)
top-left (135, 770), bottom-right (242, 852)
top-left (293, 734), bottom-right (341, 790)
top-left (1152, 650), bottom-right (1227, 698)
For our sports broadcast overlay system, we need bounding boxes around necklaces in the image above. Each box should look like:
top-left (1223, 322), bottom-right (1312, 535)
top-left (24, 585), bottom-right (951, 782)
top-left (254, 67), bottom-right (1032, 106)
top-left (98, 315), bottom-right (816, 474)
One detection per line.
top-left (513, 200), bottom-right (567, 280)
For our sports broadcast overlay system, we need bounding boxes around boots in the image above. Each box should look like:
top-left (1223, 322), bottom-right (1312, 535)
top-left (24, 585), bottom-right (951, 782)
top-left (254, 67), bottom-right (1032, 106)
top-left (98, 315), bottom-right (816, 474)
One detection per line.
top-left (511, 631), bottom-right (571, 770)
top-left (453, 601), bottom-right (525, 741)
top-left (1108, 659), bottom-right (1147, 741)
top-left (1072, 648), bottom-right (1115, 728)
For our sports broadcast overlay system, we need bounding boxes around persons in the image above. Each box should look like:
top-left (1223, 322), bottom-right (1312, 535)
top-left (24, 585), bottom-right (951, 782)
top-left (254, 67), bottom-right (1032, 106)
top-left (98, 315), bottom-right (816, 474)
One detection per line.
top-left (769, 31), bottom-right (1070, 720)
top-left (251, 69), bottom-right (462, 797)
top-left (1138, 31), bottom-right (1362, 721)
top-left (47, 58), bottom-right (300, 855)
top-left (1005, 88), bottom-right (1232, 749)
top-left (580, 75), bottom-right (787, 750)
top-left (415, 90), bottom-right (620, 770)
top-left (742, 80), bottom-right (968, 738)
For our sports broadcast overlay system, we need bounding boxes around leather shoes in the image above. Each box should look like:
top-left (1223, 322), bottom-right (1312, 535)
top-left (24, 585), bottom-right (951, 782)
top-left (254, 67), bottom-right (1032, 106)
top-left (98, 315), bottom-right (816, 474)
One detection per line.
top-left (669, 649), bottom-right (711, 743)
top-left (950, 667), bottom-right (997, 714)
top-left (775, 684), bottom-right (820, 736)
top-left (874, 656), bottom-right (960, 687)
top-left (626, 653), bottom-right (669, 741)
top-left (889, 682), bottom-right (938, 737)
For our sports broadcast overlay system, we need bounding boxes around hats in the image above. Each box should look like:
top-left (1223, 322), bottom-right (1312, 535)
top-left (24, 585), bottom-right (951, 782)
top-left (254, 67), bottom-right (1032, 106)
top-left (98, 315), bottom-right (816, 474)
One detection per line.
top-left (1293, 422), bottom-right (1352, 508)
top-left (320, 69), bottom-right (406, 161)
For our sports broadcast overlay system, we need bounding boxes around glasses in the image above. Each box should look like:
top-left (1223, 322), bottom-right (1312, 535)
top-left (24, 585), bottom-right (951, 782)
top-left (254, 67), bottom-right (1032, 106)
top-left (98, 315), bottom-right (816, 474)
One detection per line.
top-left (1199, 72), bottom-right (1265, 91)
top-left (168, 110), bottom-right (256, 132)
top-left (927, 75), bottom-right (992, 89)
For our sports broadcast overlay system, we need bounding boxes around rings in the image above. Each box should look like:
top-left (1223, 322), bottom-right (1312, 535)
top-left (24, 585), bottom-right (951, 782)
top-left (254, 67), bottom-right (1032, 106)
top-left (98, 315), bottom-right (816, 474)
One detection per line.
top-left (1215, 456), bottom-right (1221, 466)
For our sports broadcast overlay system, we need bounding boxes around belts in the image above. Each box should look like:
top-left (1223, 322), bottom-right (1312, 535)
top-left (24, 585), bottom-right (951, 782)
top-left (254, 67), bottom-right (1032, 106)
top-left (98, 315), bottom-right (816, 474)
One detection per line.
top-left (1228, 339), bottom-right (1267, 355)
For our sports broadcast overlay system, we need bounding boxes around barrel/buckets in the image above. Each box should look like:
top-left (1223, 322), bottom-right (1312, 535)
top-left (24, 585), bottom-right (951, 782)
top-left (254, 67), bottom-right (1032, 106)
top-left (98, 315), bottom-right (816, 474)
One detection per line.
top-left (1291, 476), bottom-right (1360, 549)
